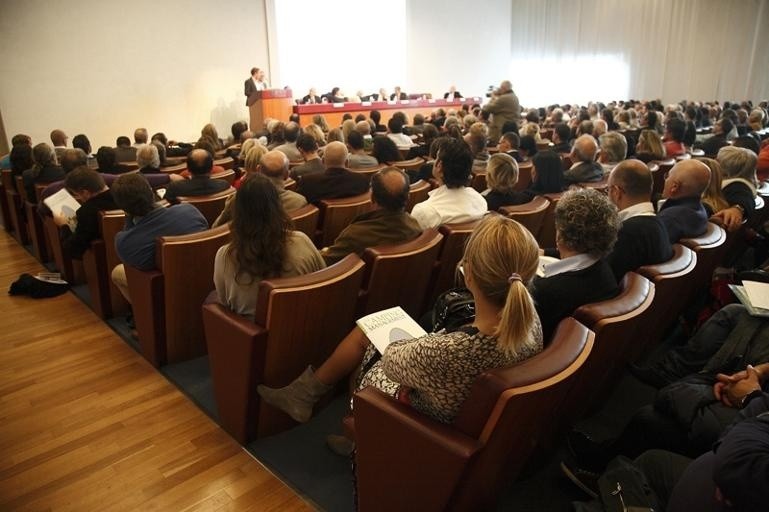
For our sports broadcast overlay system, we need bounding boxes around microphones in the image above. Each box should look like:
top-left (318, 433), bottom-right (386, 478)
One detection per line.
top-left (259, 80), bottom-right (267, 89)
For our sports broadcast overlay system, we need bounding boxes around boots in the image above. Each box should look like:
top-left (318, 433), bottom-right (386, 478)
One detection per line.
top-left (328, 434), bottom-right (350, 458)
top-left (256, 365), bottom-right (334, 424)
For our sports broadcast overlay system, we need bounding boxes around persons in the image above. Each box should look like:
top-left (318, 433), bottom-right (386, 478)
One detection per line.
top-left (552, 362), bottom-right (769, 511)
top-left (245, 67), bottom-right (267, 104)
top-left (485, 80), bottom-right (520, 138)
top-left (521, 186), bottom-right (625, 341)
top-left (2, 97), bottom-right (769, 324)
top-left (253, 216), bottom-right (549, 458)
top-left (626, 280), bottom-right (768, 386)
top-left (444, 86), bottom-right (463, 98)
top-left (302, 85), bottom-right (407, 105)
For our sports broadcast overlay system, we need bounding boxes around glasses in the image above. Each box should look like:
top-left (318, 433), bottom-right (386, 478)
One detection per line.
top-left (72, 187), bottom-right (87, 200)
top-left (663, 172), bottom-right (681, 192)
top-left (603, 185), bottom-right (625, 194)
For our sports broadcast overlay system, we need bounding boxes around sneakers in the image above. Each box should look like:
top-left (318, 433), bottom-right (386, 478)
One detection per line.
top-left (568, 428), bottom-right (594, 460)
top-left (559, 462), bottom-right (600, 500)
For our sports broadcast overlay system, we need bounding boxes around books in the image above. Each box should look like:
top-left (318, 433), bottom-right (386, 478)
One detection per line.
top-left (356, 305), bottom-right (430, 355)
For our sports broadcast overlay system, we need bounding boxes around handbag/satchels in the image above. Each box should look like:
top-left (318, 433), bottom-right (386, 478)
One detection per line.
top-left (431, 288), bottom-right (475, 336)
top-left (8, 273), bottom-right (72, 299)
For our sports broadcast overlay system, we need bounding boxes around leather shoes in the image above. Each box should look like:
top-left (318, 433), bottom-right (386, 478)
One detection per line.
top-left (626, 359), bottom-right (667, 389)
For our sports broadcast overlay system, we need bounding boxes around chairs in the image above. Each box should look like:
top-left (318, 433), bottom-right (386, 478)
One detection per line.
top-left (1, 127), bottom-right (769, 512)
